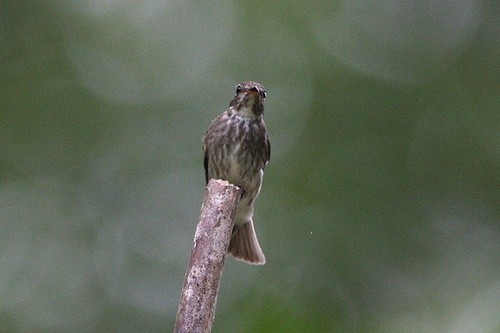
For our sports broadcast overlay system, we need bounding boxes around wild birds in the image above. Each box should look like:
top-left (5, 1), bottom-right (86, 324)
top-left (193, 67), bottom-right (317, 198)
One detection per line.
top-left (202, 80), bottom-right (271, 265)
